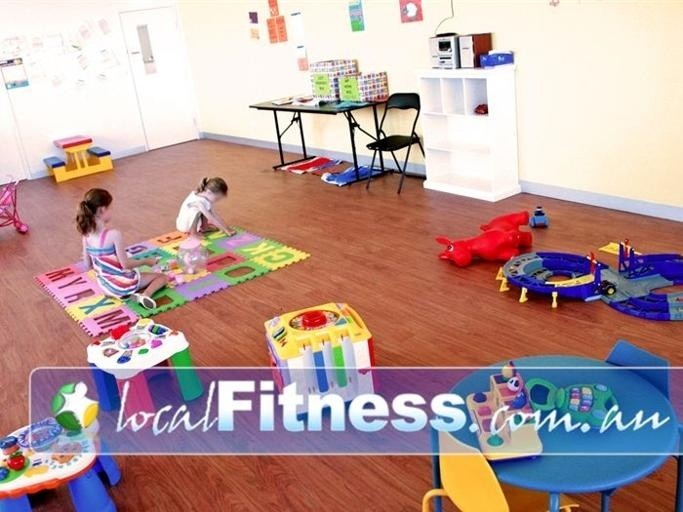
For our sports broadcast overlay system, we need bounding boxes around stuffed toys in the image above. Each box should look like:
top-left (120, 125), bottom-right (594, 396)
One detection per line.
top-left (435, 207), bottom-right (683, 331)
top-left (464, 363), bottom-right (621, 462)
top-left (264, 303), bottom-right (377, 417)
top-left (0, 379), bottom-right (121, 512)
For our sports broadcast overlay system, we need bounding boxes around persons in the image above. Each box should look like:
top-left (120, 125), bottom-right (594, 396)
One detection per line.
top-left (175, 177), bottom-right (235, 238)
top-left (75, 188), bottom-right (166, 309)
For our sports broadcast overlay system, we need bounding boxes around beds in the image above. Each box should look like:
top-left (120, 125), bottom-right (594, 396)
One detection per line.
top-left (431, 352), bottom-right (676, 512)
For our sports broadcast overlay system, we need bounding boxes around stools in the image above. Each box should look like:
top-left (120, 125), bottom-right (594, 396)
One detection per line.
top-left (1, 412), bottom-right (122, 512)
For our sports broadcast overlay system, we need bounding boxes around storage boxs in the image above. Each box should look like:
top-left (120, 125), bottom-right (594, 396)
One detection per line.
top-left (309, 57), bottom-right (358, 101)
top-left (339, 72), bottom-right (389, 103)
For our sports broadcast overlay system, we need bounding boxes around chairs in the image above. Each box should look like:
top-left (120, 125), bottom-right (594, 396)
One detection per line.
top-left (365, 93), bottom-right (424, 194)
top-left (419, 428), bottom-right (582, 508)
top-left (592, 339), bottom-right (682, 507)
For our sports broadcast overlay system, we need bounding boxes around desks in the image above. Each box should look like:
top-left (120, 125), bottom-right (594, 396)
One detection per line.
top-left (249, 97), bottom-right (384, 183)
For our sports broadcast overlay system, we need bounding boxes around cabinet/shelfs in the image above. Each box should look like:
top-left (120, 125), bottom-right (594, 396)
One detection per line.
top-left (417, 67), bottom-right (521, 203)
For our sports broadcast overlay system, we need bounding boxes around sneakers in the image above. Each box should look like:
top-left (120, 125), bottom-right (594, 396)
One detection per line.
top-left (130, 292), bottom-right (157, 311)
